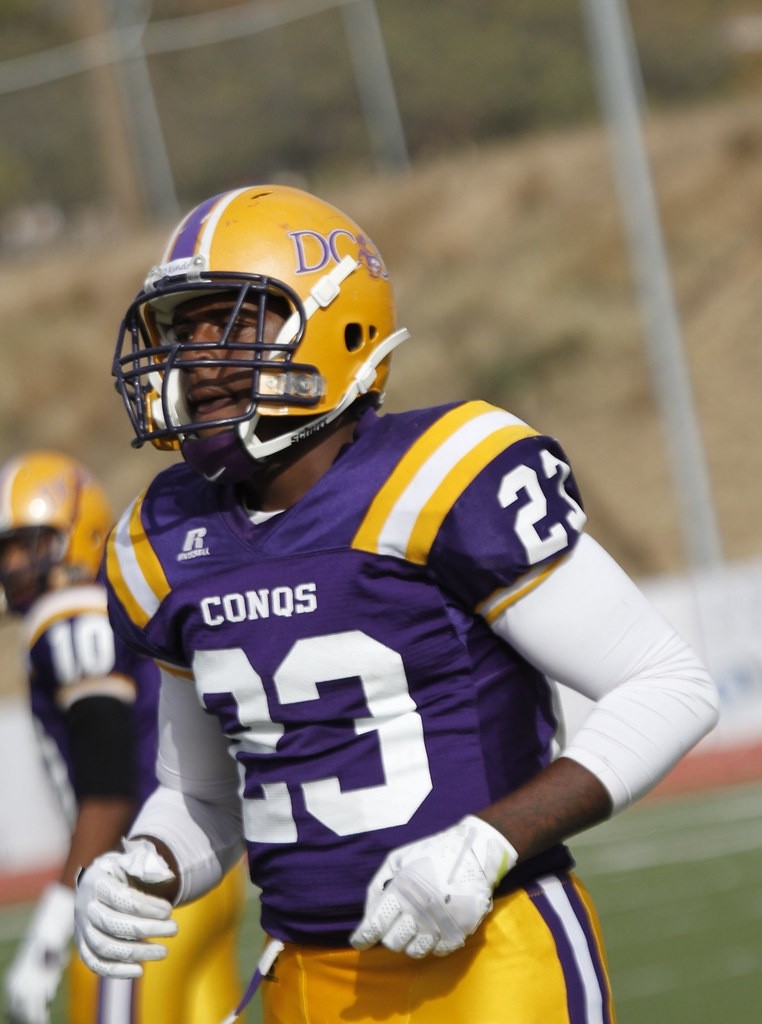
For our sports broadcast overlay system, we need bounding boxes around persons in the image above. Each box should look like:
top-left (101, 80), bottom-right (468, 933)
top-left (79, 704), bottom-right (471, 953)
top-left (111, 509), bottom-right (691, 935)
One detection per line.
top-left (74, 185), bottom-right (721, 1024)
top-left (0, 451), bottom-right (247, 1024)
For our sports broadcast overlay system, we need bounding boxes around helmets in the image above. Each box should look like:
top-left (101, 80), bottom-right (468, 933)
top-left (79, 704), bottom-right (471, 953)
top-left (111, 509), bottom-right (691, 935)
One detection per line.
top-left (0, 451), bottom-right (122, 599)
top-left (114, 183), bottom-right (398, 450)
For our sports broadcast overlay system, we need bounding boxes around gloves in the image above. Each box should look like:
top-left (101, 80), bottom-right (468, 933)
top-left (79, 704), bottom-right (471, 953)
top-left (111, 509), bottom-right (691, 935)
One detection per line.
top-left (73, 838), bottom-right (179, 981)
top-left (351, 813), bottom-right (520, 961)
top-left (6, 881), bottom-right (80, 1024)
